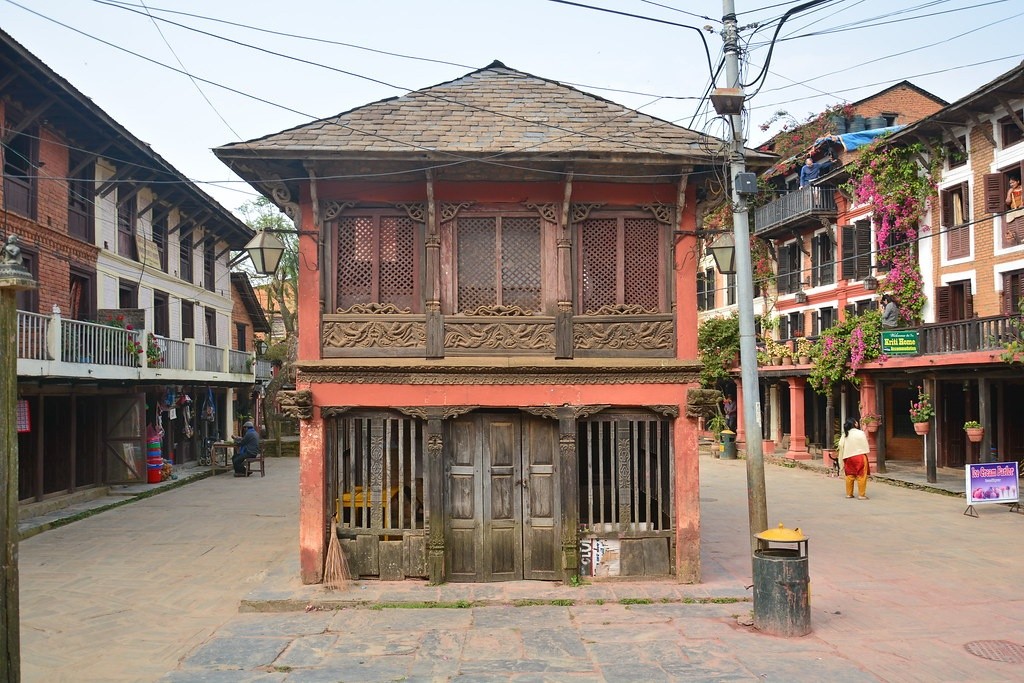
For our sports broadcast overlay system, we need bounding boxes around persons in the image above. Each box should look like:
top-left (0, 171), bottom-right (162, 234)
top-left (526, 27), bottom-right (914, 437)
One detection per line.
top-left (881, 295), bottom-right (898, 330)
top-left (233, 422), bottom-right (258, 476)
top-left (724, 394), bottom-right (736, 427)
top-left (838, 418), bottom-right (870, 499)
top-left (799, 157), bottom-right (837, 208)
top-left (1006, 176), bottom-right (1024, 209)
top-left (0, 235), bottom-right (23, 264)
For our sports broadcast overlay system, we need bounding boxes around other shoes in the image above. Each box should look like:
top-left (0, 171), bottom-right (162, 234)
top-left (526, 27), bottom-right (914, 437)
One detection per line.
top-left (846, 495), bottom-right (855, 498)
top-left (858, 495), bottom-right (869, 499)
top-left (234, 472), bottom-right (246, 476)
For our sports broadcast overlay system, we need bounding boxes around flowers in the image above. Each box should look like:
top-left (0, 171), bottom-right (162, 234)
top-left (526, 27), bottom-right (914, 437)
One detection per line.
top-left (861, 411), bottom-right (882, 424)
top-left (765, 338), bottom-right (814, 356)
top-left (99, 313), bottom-right (166, 365)
top-left (909, 385), bottom-right (936, 422)
top-left (963, 421), bottom-right (983, 430)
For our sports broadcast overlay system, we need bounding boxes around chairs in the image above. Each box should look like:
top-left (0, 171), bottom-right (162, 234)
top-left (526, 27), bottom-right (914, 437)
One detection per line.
top-left (246, 440), bottom-right (265, 477)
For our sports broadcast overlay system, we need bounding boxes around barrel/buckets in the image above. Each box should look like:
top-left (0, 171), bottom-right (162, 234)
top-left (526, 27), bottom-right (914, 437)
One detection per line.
top-left (147, 437), bottom-right (162, 483)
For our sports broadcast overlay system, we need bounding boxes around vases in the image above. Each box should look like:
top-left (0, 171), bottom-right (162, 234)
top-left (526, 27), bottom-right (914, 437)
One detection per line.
top-left (782, 357), bottom-right (792, 366)
top-left (967, 428), bottom-right (983, 441)
top-left (772, 357), bottom-right (781, 365)
top-left (799, 357), bottom-right (809, 364)
top-left (866, 421), bottom-right (880, 432)
top-left (914, 422), bottom-right (930, 435)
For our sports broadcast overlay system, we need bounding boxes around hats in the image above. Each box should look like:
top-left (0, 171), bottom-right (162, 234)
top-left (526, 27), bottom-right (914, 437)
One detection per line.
top-left (242, 421), bottom-right (253, 426)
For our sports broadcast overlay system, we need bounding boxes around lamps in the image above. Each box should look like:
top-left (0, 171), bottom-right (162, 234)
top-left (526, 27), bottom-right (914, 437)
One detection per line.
top-left (795, 276), bottom-right (810, 303)
top-left (864, 265), bottom-right (880, 290)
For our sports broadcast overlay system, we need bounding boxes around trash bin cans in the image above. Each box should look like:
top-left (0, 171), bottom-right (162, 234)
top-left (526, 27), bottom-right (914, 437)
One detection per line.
top-left (720, 430), bottom-right (737, 460)
top-left (751, 522), bottom-right (812, 637)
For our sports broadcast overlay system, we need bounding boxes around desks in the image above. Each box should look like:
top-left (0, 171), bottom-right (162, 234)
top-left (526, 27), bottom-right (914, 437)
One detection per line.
top-left (210, 443), bottom-right (240, 475)
